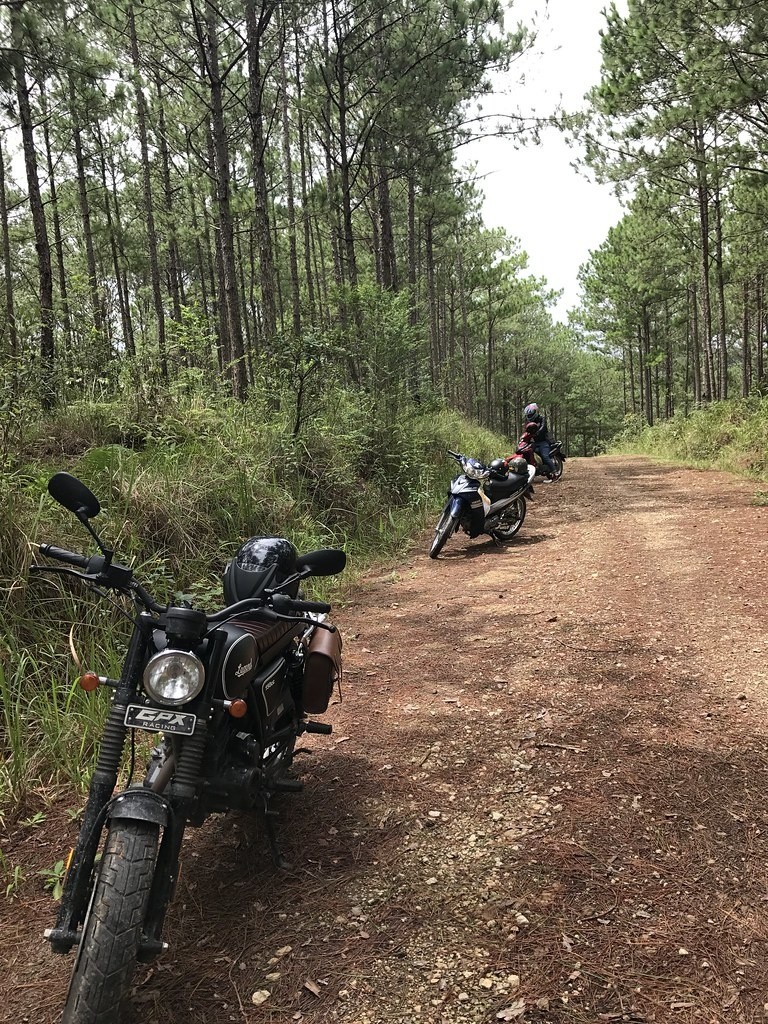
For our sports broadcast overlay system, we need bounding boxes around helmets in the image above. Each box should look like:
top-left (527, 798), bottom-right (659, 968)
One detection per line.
top-left (524, 403), bottom-right (539, 422)
top-left (507, 457), bottom-right (528, 475)
top-left (488, 459), bottom-right (510, 481)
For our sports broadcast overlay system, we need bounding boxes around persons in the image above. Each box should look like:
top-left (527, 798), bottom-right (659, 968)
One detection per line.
top-left (522, 402), bottom-right (560, 482)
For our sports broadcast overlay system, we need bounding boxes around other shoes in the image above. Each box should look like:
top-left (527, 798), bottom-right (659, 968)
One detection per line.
top-left (552, 470), bottom-right (557, 482)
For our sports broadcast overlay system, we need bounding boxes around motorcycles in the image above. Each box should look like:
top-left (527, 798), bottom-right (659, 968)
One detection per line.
top-left (515, 425), bottom-right (567, 482)
top-left (429, 447), bottom-right (536, 561)
top-left (34, 471), bottom-right (349, 1023)
